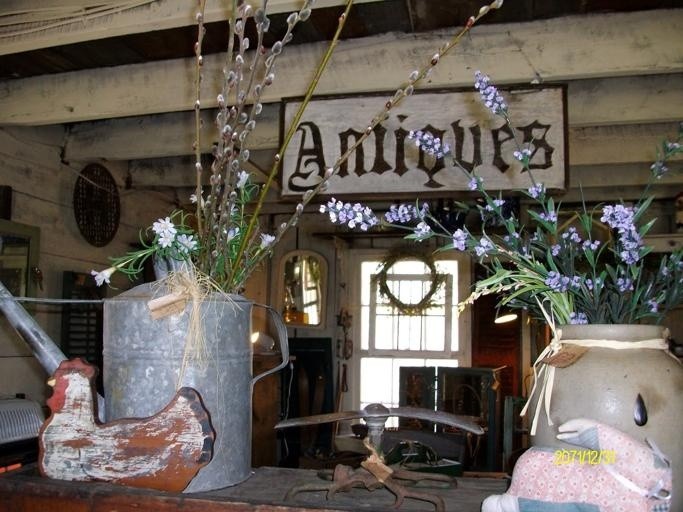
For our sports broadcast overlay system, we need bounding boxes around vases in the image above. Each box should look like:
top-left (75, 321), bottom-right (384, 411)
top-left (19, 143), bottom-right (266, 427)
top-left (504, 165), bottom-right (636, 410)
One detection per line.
top-left (103, 231), bottom-right (252, 498)
top-left (528, 324), bottom-right (682, 511)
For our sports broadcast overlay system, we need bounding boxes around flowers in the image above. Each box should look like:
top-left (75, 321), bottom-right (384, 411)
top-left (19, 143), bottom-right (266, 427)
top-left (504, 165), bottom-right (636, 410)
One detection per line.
top-left (319, 69), bottom-right (683, 324)
top-left (90, 0), bottom-right (505, 292)
top-left (370, 246), bottom-right (444, 318)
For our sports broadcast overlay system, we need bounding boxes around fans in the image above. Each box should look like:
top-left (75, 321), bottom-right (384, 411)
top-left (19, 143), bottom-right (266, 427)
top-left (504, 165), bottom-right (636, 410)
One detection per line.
top-left (276, 402), bottom-right (483, 512)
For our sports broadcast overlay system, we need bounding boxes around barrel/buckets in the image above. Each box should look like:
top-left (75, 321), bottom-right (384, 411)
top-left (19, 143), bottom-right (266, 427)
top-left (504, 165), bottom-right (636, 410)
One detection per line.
top-left (0, 243), bottom-right (291, 495)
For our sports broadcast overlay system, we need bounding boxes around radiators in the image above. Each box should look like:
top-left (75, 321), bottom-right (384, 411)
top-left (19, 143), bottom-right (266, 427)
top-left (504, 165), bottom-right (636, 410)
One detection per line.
top-left (0, 400), bottom-right (45, 444)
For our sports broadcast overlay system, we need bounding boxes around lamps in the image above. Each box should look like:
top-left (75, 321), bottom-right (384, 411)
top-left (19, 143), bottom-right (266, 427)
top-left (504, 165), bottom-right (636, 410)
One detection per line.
top-left (253, 332), bottom-right (276, 356)
top-left (494, 293), bottom-right (538, 323)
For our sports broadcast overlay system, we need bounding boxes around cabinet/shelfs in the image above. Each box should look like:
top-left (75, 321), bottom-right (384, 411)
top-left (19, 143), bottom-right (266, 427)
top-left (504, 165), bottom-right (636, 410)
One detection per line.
top-left (252, 357), bottom-right (296, 467)
top-left (0, 462), bottom-right (510, 512)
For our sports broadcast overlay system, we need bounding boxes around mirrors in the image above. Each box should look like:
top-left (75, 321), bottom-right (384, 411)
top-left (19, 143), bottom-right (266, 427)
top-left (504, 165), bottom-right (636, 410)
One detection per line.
top-left (0, 221), bottom-right (40, 315)
top-left (282, 255), bottom-right (320, 325)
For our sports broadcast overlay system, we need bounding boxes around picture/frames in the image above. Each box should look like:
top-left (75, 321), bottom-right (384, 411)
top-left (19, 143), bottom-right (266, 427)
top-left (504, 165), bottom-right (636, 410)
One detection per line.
top-left (3, 240), bottom-right (27, 255)
top-left (530, 320), bottom-right (553, 367)
top-left (0, 256), bottom-right (25, 303)
